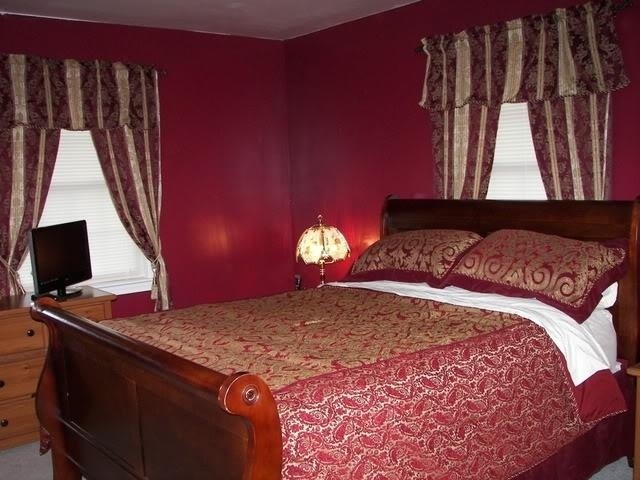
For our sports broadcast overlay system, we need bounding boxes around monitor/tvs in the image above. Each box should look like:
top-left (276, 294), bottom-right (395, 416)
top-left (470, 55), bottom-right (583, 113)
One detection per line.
top-left (30, 219), bottom-right (92, 302)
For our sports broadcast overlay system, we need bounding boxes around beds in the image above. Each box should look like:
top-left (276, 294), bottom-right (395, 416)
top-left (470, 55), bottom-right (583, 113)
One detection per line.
top-left (431, 226), bottom-right (630, 325)
top-left (28, 192), bottom-right (639, 480)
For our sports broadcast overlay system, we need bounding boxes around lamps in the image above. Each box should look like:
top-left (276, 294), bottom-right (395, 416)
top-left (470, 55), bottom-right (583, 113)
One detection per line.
top-left (294, 213), bottom-right (351, 287)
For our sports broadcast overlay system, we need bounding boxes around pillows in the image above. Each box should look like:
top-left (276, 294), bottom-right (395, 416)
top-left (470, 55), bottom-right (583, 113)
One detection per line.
top-left (340, 228), bottom-right (485, 288)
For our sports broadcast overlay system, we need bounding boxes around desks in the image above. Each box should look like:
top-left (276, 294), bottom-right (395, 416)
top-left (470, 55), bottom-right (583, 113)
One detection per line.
top-left (626, 364), bottom-right (639, 480)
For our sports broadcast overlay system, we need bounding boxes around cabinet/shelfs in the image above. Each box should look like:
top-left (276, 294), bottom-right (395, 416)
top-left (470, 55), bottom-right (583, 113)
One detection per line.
top-left (0, 284), bottom-right (117, 449)
